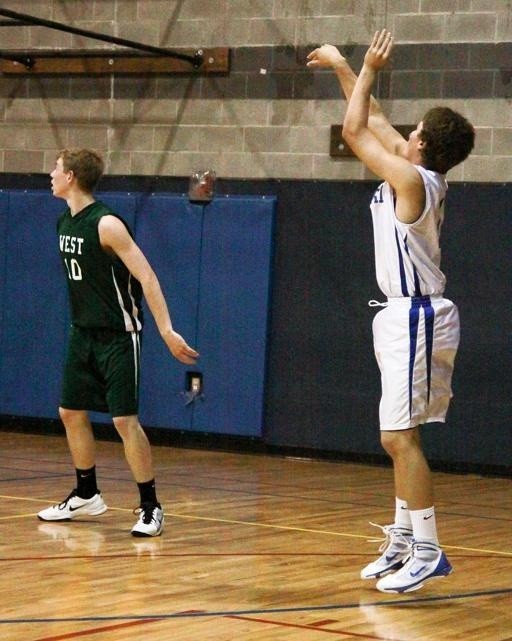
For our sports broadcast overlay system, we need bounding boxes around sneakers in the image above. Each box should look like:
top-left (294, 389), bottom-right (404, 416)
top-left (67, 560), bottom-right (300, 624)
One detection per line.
top-left (36, 491), bottom-right (107, 520)
top-left (361, 527), bottom-right (453, 594)
top-left (131, 504), bottom-right (164, 537)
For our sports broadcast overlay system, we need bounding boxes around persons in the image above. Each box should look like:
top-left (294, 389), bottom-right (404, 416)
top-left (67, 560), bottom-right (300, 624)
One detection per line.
top-left (305, 25), bottom-right (476, 596)
top-left (36, 147), bottom-right (200, 537)
